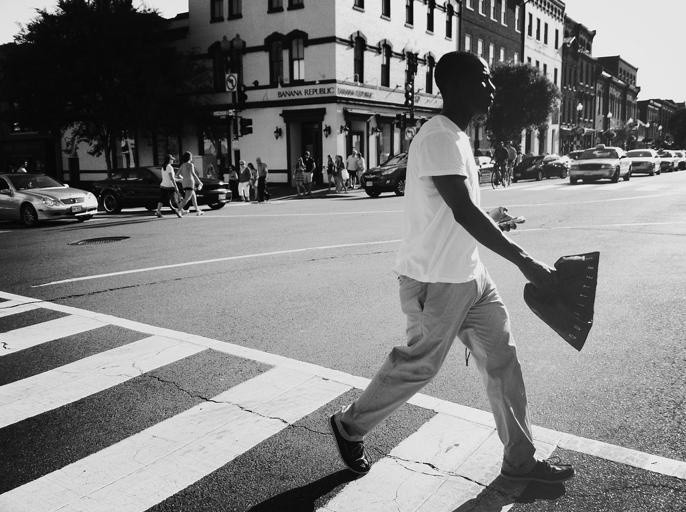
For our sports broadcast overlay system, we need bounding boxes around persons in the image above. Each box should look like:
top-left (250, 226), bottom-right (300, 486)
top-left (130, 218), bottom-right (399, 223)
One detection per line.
top-left (155, 154), bottom-right (189, 217)
top-left (506, 141), bottom-right (518, 179)
top-left (174, 151), bottom-right (204, 218)
top-left (491, 141), bottom-right (510, 179)
top-left (18, 161), bottom-right (28, 173)
top-left (331, 52), bottom-right (575, 483)
top-left (228, 150), bottom-right (366, 202)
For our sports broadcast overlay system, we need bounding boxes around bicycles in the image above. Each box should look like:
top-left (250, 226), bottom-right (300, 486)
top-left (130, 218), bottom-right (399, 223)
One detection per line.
top-left (490, 162), bottom-right (515, 189)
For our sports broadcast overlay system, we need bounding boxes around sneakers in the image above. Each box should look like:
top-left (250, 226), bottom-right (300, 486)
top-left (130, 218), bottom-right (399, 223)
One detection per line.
top-left (175, 208), bottom-right (183, 218)
top-left (196, 211), bottom-right (203, 216)
top-left (328, 413), bottom-right (373, 476)
top-left (500, 458), bottom-right (575, 485)
top-left (154, 211), bottom-right (163, 218)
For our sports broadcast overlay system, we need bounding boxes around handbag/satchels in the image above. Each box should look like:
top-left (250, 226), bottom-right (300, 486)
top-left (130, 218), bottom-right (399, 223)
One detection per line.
top-left (340, 168), bottom-right (349, 180)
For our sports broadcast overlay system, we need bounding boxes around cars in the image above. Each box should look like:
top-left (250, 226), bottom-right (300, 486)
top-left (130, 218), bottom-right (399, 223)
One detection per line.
top-left (655, 149), bottom-right (686, 172)
top-left (358, 152), bottom-right (408, 197)
top-left (474, 156), bottom-right (494, 185)
top-left (96, 162), bottom-right (234, 215)
top-left (625, 148), bottom-right (662, 180)
top-left (509, 153), bottom-right (568, 182)
top-left (568, 144), bottom-right (633, 181)
top-left (0, 167), bottom-right (99, 230)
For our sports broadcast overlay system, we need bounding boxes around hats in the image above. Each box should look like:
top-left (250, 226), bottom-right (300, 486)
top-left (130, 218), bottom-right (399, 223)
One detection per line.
top-left (165, 154), bottom-right (174, 160)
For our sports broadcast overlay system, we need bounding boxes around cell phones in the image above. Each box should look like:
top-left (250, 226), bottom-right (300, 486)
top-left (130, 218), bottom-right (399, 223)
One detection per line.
top-left (497, 215), bottom-right (527, 229)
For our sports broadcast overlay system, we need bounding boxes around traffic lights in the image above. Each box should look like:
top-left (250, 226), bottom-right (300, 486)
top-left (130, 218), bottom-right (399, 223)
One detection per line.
top-left (239, 118), bottom-right (254, 135)
top-left (404, 80), bottom-right (414, 108)
